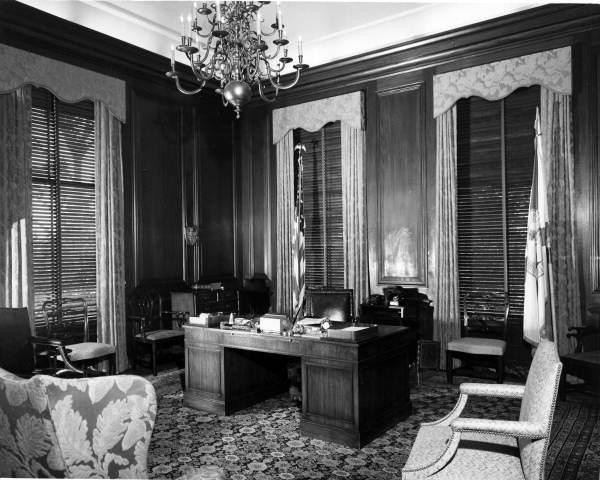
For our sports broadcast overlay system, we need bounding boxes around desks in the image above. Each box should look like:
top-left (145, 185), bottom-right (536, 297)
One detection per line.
top-left (181, 307), bottom-right (411, 449)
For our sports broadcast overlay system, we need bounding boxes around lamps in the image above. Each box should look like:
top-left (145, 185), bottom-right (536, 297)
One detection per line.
top-left (162, 2), bottom-right (309, 119)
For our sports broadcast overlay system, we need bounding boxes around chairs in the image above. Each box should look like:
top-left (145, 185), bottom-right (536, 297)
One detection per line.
top-left (125, 282), bottom-right (191, 375)
top-left (304, 286), bottom-right (356, 322)
top-left (446, 286), bottom-right (508, 386)
top-left (0, 297), bottom-right (159, 479)
top-left (398, 323), bottom-right (600, 480)
